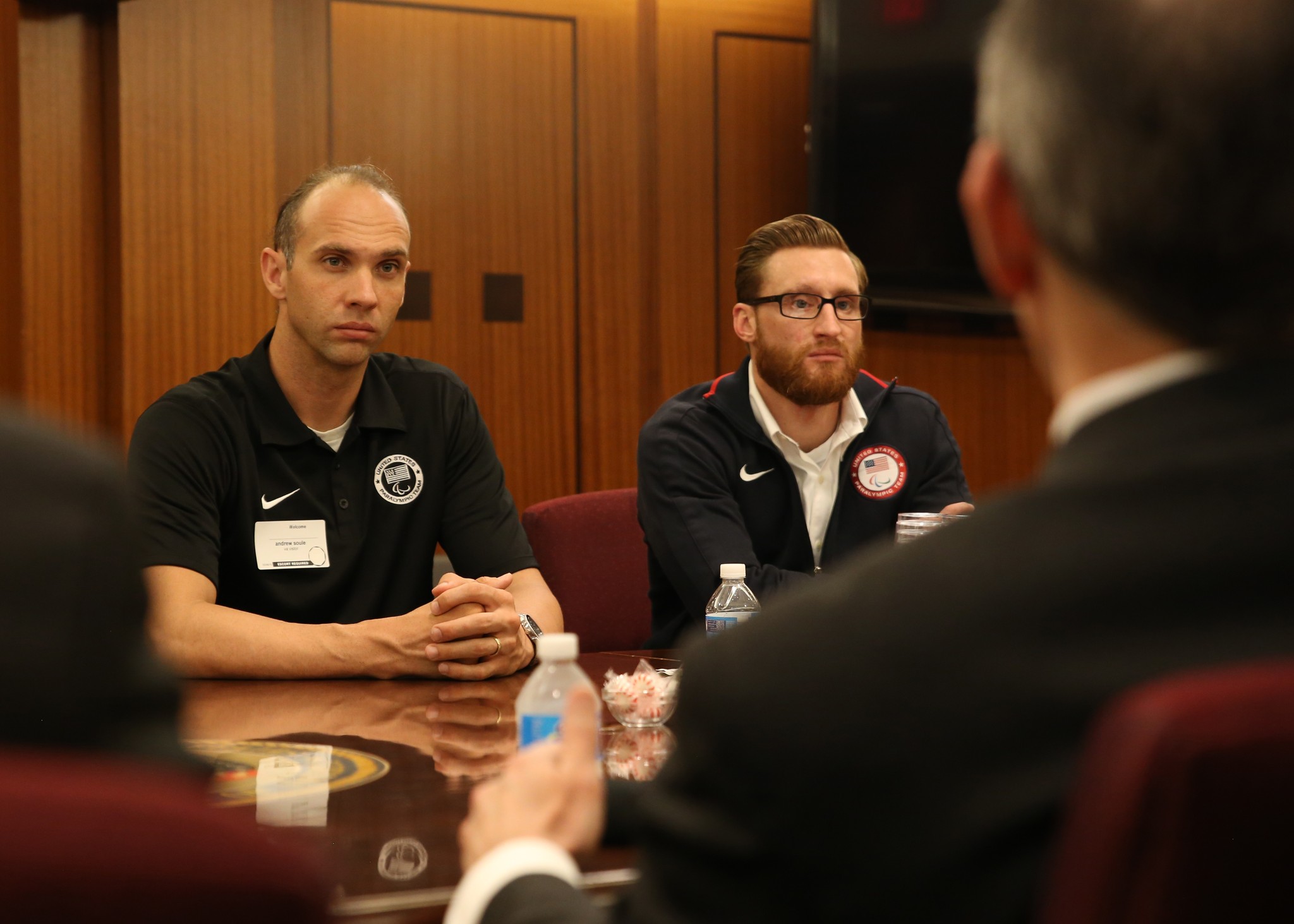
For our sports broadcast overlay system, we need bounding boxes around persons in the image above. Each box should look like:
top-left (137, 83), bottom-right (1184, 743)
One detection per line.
top-left (0, 413), bottom-right (218, 924)
top-left (122, 164), bottom-right (566, 684)
top-left (633, 214), bottom-right (978, 647)
top-left (175, 673), bottom-right (533, 912)
top-left (437, 0), bottom-right (1293, 924)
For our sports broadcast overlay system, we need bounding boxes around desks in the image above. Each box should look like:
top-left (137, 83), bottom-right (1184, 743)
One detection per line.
top-left (162, 636), bottom-right (685, 924)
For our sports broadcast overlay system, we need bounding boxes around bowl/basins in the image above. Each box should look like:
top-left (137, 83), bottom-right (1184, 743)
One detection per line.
top-left (601, 688), bottom-right (676, 727)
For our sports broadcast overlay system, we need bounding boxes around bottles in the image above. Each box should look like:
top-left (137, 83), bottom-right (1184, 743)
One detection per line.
top-left (705, 564), bottom-right (762, 640)
top-left (514, 632), bottom-right (600, 768)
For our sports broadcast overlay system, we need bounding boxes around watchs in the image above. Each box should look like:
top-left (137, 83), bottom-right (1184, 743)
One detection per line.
top-left (519, 614), bottom-right (544, 643)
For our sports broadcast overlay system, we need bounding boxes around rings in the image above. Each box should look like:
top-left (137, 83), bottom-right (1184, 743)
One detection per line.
top-left (492, 705), bottom-right (504, 727)
top-left (489, 636), bottom-right (502, 657)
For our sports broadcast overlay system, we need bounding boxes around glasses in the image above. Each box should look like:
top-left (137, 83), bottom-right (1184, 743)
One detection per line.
top-left (746, 293), bottom-right (871, 321)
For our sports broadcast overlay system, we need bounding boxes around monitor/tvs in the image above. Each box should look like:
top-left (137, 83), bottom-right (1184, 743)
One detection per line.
top-left (805, 1), bottom-right (1024, 341)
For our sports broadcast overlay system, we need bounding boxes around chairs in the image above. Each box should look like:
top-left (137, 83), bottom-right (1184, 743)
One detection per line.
top-left (520, 483), bottom-right (661, 652)
